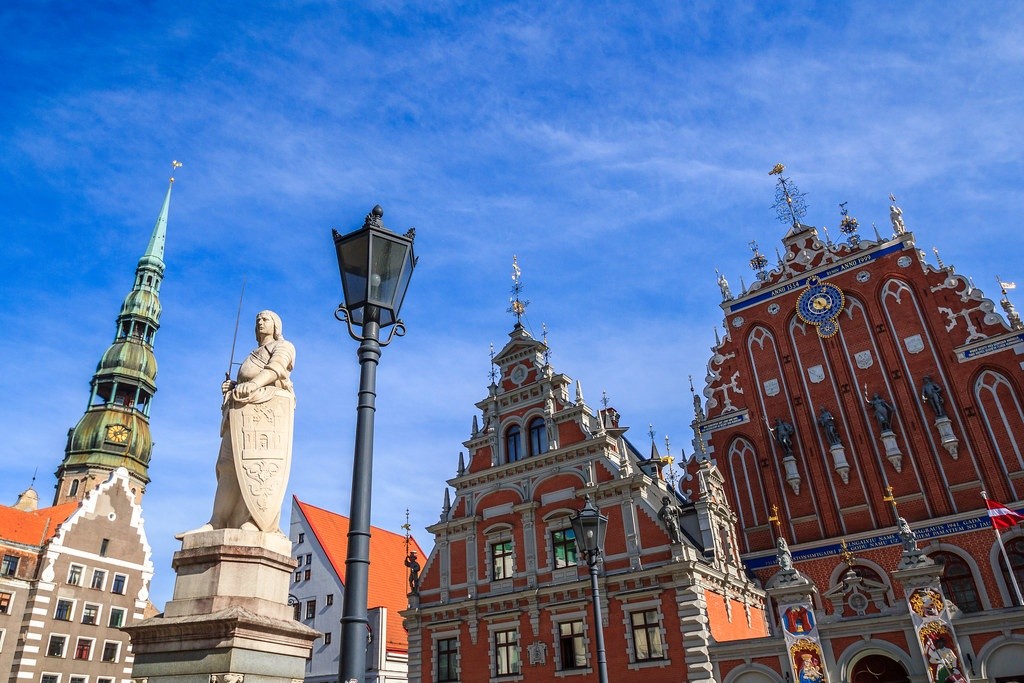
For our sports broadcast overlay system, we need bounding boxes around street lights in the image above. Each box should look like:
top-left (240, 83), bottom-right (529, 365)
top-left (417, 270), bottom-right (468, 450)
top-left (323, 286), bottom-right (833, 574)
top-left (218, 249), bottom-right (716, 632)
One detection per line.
top-left (330, 203), bottom-right (420, 683)
top-left (567, 492), bottom-right (611, 682)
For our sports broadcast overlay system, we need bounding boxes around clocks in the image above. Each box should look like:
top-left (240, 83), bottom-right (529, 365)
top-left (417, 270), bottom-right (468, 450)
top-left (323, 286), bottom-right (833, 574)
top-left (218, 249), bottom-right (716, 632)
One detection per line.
top-left (795, 275), bottom-right (846, 339)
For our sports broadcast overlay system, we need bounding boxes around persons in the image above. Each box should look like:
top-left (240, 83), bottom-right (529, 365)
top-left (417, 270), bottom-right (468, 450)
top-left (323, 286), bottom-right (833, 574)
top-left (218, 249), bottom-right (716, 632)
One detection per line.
top-left (404, 556), bottom-right (419, 592)
top-left (865, 392), bottom-right (895, 432)
top-left (921, 376), bottom-right (945, 417)
top-left (890, 206), bottom-right (905, 235)
top-left (657, 496), bottom-right (683, 544)
top-left (175, 310), bottom-right (295, 541)
top-left (817, 405), bottom-right (838, 445)
top-left (717, 275), bottom-right (730, 302)
top-left (768, 418), bottom-right (794, 457)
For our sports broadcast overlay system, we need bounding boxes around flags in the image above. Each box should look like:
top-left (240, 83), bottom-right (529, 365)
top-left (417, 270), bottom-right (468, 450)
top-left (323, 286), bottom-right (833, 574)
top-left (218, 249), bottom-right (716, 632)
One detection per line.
top-left (983, 498), bottom-right (1024, 530)
